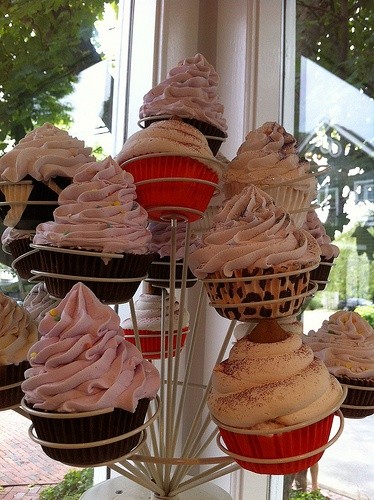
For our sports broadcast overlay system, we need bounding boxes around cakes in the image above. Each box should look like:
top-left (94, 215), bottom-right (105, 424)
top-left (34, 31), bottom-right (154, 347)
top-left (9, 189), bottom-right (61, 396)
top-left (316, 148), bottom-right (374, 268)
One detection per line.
top-left (206, 319), bottom-right (344, 475)
top-left (0, 122), bottom-right (98, 230)
top-left (187, 184), bottom-right (322, 322)
top-left (139, 52), bottom-right (228, 157)
top-left (121, 285), bottom-right (189, 360)
top-left (31, 155), bottom-right (152, 304)
top-left (114, 119), bottom-right (224, 221)
top-left (0, 224), bottom-right (42, 280)
top-left (22, 282), bottom-right (62, 325)
top-left (146, 120), bottom-right (339, 291)
top-left (300, 311), bottom-right (374, 419)
top-left (0, 289), bottom-right (41, 410)
top-left (20, 282), bottom-right (163, 466)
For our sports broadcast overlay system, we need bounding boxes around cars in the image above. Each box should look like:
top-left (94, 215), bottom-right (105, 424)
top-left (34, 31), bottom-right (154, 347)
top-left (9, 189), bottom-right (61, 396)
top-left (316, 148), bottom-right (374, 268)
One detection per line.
top-left (338, 297), bottom-right (372, 311)
